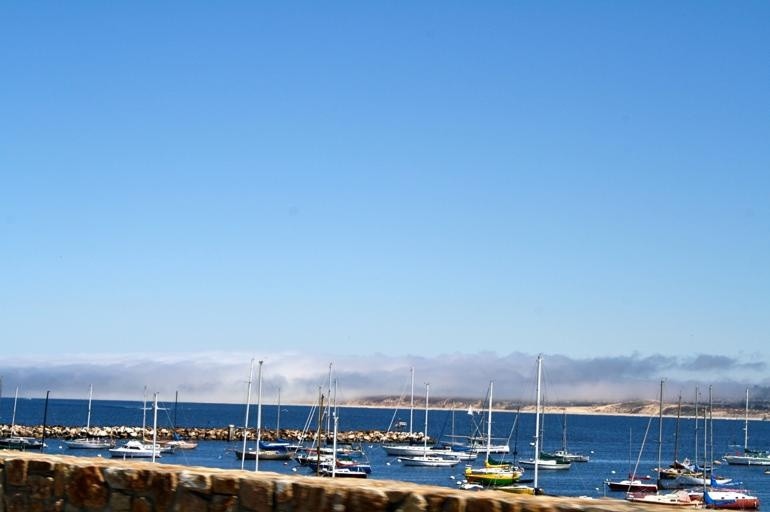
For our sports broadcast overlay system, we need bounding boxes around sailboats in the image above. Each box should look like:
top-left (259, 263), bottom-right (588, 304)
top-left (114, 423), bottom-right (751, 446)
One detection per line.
top-left (380, 352), bottom-right (591, 496)
top-left (60, 383), bottom-right (117, 449)
top-left (234, 356), bottom-right (373, 479)
top-left (604, 428), bottom-right (657, 493)
top-left (0, 384), bottom-right (50, 450)
top-left (720, 387), bottom-right (770, 465)
top-left (110, 385), bottom-right (199, 458)
top-left (624, 379), bottom-right (760, 511)
